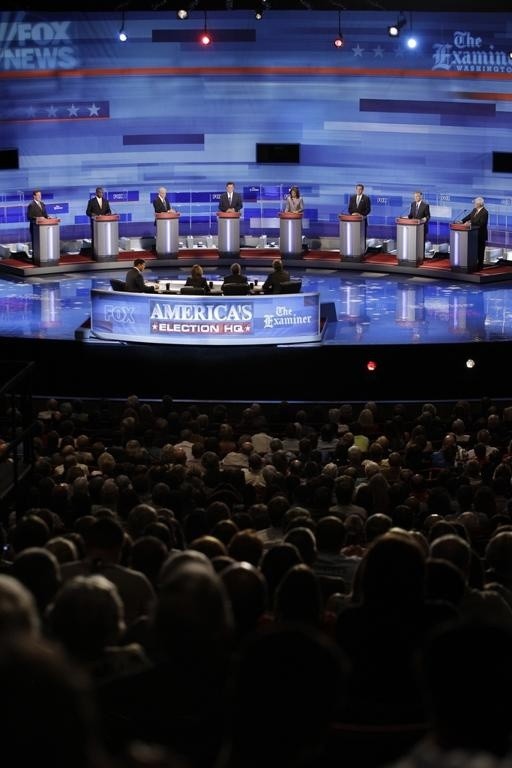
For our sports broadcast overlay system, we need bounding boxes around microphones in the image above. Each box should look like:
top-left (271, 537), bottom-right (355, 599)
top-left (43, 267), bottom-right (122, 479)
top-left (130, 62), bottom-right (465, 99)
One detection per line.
top-left (341, 200), bottom-right (354, 215)
top-left (454, 209), bottom-right (467, 224)
top-left (44, 205), bottom-right (57, 219)
top-left (399, 205), bottom-right (413, 218)
top-left (229, 197), bottom-right (238, 212)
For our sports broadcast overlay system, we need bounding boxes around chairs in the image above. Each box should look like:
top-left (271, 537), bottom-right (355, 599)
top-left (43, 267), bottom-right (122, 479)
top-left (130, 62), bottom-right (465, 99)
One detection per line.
top-left (221, 462), bottom-right (248, 470)
top-left (221, 283), bottom-right (250, 296)
top-left (276, 280), bottom-right (302, 293)
top-left (110, 279), bottom-right (125, 292)
top-left (179, 286), bottom-right (205, 295)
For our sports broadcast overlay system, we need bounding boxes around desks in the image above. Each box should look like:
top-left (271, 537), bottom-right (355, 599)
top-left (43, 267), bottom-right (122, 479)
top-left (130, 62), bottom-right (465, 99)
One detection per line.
top-left (143, 280), bottom-right (267, 294)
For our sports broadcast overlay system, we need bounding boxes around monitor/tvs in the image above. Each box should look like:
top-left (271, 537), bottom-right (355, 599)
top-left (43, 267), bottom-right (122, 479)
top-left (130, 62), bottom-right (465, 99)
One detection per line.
top-left (0, 148), bottom-right (20, 170)
top-left (491, 150), bottom-right (512, 178)
top-left (256, 143), bottom-right (302, 163)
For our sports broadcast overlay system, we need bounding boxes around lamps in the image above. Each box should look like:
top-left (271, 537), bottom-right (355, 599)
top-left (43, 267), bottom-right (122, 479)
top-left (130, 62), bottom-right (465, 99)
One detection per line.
top-left (255, 0), bottom-right (266, 20)
top-left (118, 4), bottom-right (128, 42)
top-left (406, 11), bottom-right (416, 49)
top-left (387, 10), bottom-right (406, 37)
top-left (177, 0), bottom-right (191, 19)
top-left (198, 11), bottom-right (212, 48)
top-left (332, 6), bottom-right (344, 48)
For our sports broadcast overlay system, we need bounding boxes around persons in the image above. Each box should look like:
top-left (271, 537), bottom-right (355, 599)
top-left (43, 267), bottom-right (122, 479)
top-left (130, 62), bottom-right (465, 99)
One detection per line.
top-left (262, 258), bottom-right (290, 294)
top-left (86, 186), bottom-right (111, 260)
top-left (184, 263), bottom-right (211, 295)
top-left (454, 197), bottom-right (489, 273)
top-left (403, 192), bottom-right (430, 261)
top-left (126, 258), bottom-right (159, 293)
top-left (1, 390), bottom-right (511, 766)
top-left (348, 184), bottom-right (370, 249)
top-left (153, 187), bottom-right (176, 252)
top-left (220, 263), bottom-right (248, 295)
top-left (26, 190), bottom-right (54, 264)
top-left (218, 181), bottom-right (242, 213)
top-left (284, 186), bottom-right (304, 213)
